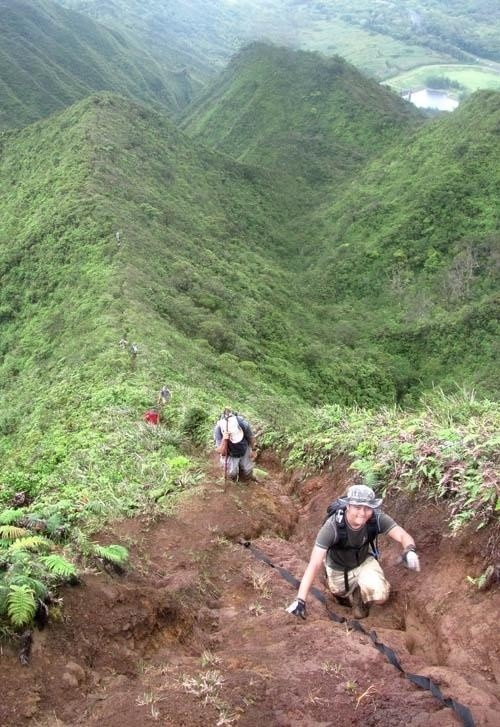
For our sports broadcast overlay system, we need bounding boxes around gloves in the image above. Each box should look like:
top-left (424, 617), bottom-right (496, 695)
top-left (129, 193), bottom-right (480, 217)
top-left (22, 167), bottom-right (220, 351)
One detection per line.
top-left (395, 547), bottom-right (421, 573)
top-left (286, 597), bottom-right (307, 620)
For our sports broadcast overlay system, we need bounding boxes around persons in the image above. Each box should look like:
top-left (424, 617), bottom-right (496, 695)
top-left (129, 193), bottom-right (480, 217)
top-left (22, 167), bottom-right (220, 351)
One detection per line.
top-left (144, 409), bottom-right (160, 424)
top-left (285, 485), bottom-right (420, 620)
top-left (157, 387), bottom-right (170, 403)
top-left (214, 408), bottom-right (257, 482)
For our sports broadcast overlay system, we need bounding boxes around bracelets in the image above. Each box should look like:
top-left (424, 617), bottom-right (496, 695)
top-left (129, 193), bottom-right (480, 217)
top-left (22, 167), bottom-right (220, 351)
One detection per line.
top-left (252, 449), bottom-right (257, 451)
top-left (404, 544), bottom-right (417, 554)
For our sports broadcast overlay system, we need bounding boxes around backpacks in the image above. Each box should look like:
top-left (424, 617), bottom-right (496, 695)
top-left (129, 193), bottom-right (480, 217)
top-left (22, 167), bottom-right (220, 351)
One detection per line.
top-left (323, 499), bottom-right (380, 550)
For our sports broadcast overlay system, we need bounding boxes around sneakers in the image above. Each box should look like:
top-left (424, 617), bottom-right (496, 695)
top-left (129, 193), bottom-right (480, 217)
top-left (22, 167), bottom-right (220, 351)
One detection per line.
top-left (336, 594), bottom-right (352, 607)
top-left (350, 588), bottom-right (368, 619)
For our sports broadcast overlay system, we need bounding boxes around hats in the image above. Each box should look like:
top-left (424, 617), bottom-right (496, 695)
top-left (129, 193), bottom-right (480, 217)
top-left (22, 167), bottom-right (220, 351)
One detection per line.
top-left (221, 416), bottom-right (244, 443)
top-left (338, 482), bottom-right (383, 509)
